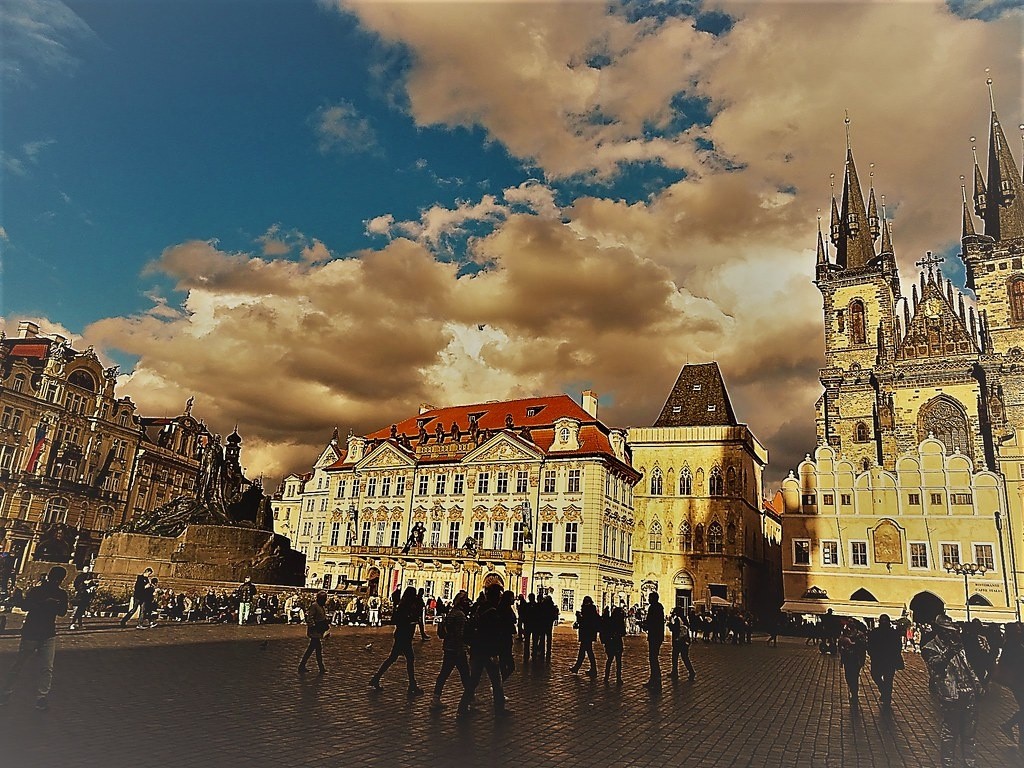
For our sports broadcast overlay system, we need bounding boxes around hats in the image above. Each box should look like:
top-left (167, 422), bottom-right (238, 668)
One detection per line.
top-left (935, 615), bottom-right (957, 633)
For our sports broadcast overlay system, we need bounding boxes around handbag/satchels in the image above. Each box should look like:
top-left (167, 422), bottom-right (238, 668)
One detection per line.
top-left (317, 620), bottom-right (329, 634)
top-left (675, 616), bottom-right (688, 640)
top-left (461, 605), bottom-right (497, 646)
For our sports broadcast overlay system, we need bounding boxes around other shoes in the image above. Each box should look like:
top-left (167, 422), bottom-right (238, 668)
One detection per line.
top-left (666, 672), bottom-right (679, 679)
top-left (616, 680), bottom-right (623, 683)
top-left (136, 625), bottom-right (146, 630)
top-left (429, 701), bottom-right (449, 710)
top-left (149, 623), bottom-right (157, 628)
top-left (496, 709), bottom-right (516, 715)
top-left (298, 664), bottom-right (309, 671)
top-left (468, 697), bottom-right (483, 706)
top-left (407, 686), bottom-right (424, 694)
top-left (69, 625), bottom-right (76, 629)
top-left (605, 678), bottom-right (607, 681)
top-left (568, 667), bottom-right (578, 673)
top-left (689, 672), bottom-right (695, 680)
top-left (643, 682), bottom-right (662, 691)
top-left (78, 626), bottom-right (86, 630)
top-left (33, 698), bottom-right (46, 710)
top-left (321, 667), bottom-right (328, 672)
top-left (585, 670), bottom-right (597, 677)
top-left (1, 689), bottom-right (15, 705)
top-left (368, 678), bottom-right (383, 689)
top-left (456, 708), bottom-right (480, 715)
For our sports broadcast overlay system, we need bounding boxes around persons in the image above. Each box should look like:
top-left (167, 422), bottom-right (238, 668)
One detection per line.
top-left (299, 592), bottom-right (330, 673)
top-left (0, 567), bottom-right (67, 709)
top-left (68, 566), bottom-right (1024, 768)
